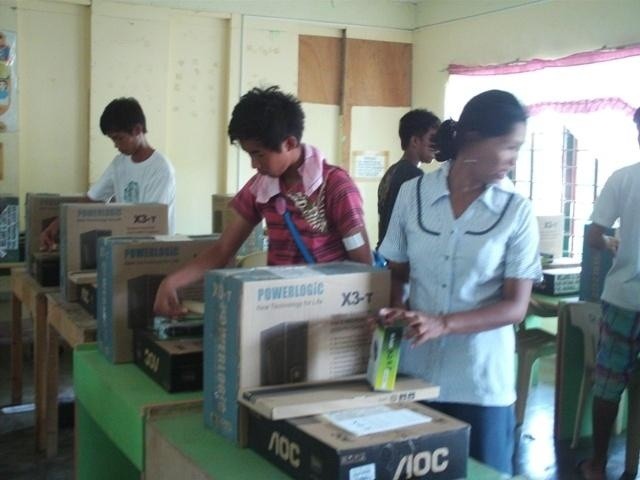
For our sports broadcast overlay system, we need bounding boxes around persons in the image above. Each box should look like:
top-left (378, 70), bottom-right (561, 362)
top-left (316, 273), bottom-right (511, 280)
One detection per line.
top-left (378, 109), bottom-right (442, 249)
top-left (365, 91), bottom-right (544, 476)
top-left (153, 85), bottom-right (372, 316)
top-left (576, 108), bottom-right (639, 479)
top-left (40, 96), bottom-right (176, 249)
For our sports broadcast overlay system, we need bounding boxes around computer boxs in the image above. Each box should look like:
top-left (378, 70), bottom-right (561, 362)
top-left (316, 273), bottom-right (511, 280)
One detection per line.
top-left (258, 320), bottom-right (309, 384)
top-left (79, 229), bottom-right (112, 270)
top-left (127, 273), bottom-right (165, 328)
